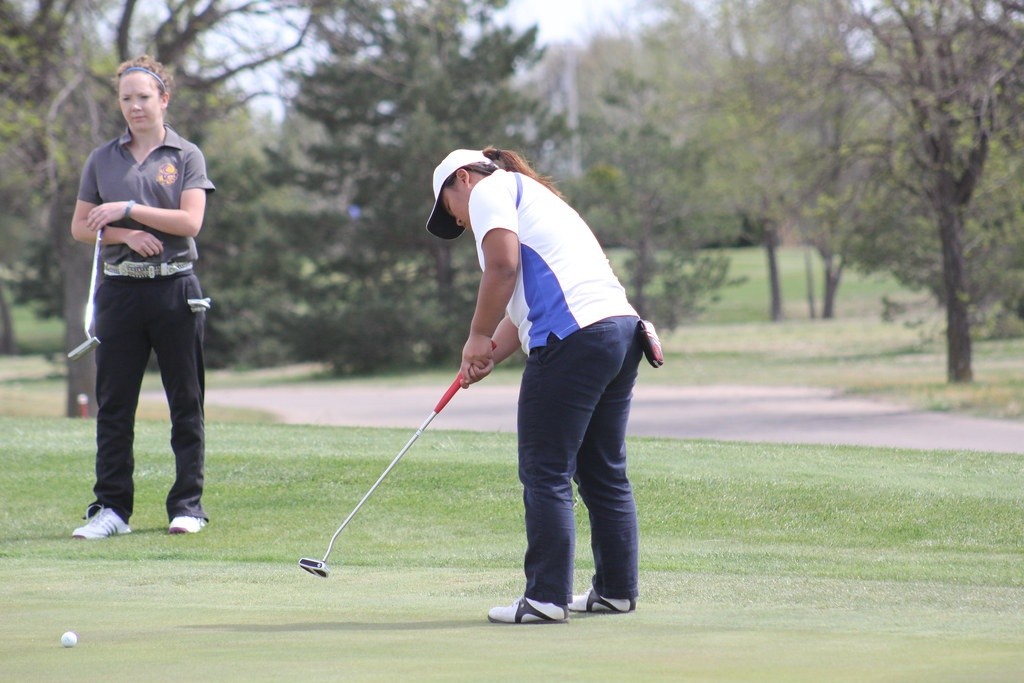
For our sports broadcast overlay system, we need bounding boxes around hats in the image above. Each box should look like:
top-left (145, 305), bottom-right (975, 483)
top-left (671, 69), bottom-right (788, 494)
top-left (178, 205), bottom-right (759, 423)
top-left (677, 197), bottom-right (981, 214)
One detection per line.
top-left (426, 149), bottom-right (499, 241)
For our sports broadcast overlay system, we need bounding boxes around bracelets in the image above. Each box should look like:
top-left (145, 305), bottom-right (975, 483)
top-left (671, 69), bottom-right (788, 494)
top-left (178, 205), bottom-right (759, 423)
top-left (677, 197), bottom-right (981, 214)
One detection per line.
top-left (124, 199), bottom-right (135, 218)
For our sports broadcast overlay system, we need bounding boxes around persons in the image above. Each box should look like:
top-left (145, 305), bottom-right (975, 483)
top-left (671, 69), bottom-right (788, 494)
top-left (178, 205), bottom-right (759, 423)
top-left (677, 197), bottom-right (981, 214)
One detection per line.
top-left (71, 57), bottom-right (215, 542)
top-left (425, 145), bottom-right (662, 623)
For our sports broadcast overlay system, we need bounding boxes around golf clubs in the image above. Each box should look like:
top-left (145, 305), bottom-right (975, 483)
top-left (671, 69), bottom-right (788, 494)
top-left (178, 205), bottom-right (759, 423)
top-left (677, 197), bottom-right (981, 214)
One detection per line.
top-left (66, 228), bottom-right (103, 362)
top-left (297, 339), bottom-right (498, 580)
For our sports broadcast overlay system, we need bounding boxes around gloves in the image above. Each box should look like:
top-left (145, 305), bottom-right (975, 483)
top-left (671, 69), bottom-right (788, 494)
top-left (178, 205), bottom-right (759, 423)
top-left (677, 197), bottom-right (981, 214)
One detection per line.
top-left (188, 297), bottom-right (211, 313)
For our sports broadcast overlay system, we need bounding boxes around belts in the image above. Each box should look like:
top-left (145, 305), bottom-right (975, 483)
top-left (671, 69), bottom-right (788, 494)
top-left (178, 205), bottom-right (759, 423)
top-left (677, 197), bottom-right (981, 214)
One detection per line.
top-left (103, 260), bottom-right (192, 278)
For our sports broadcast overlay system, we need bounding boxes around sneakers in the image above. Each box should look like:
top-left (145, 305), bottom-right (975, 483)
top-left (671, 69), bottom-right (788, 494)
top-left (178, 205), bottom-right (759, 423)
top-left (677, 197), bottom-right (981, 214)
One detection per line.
top-left (168, 515), bottom-right (206, 533)
top-left (72, 504), bottom-right (131, 538)
top-left (568, 587), bottom-right (636, 613)
top-left (488, 595), bottom-right (569, 622)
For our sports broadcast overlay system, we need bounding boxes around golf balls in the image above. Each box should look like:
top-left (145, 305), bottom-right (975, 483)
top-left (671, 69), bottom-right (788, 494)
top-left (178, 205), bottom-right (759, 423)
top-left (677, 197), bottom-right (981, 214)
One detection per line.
top-left (60, 631), bottom-right (79, 648)
top-left (76, 392), bottom-right (90, 406)
